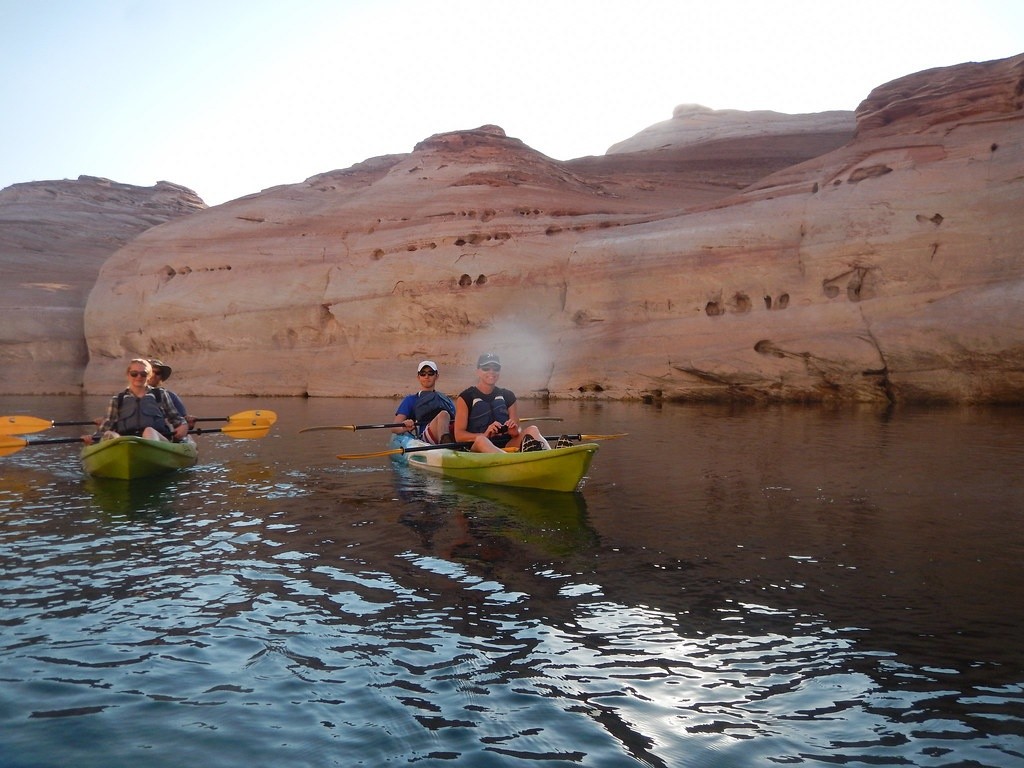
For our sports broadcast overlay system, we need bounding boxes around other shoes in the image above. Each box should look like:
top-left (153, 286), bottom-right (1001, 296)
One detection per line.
top-left (555, 434), bottom-right (574, 450)
top-left (440, 433), bottom-right (453, 444)
top-left (520, 434), bottom-right (543, 453)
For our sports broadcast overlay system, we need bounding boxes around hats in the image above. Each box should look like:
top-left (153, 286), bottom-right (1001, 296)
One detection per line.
top-left (418, 361), bottom-right (438, 373)
top-left (477, 353), bottom-right (501, 367)
top-left (147, 359), bottom-right (172, 382)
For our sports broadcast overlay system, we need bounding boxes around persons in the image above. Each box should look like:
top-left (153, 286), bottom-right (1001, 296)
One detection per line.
top-left (454, 352), bottom-right (551, 453)
top-left (389, 360), bottom-right (456, 446)
top-left (81, 358), bottom-right (189, 446)
top-left (95, 359), bottom-right (196, 430)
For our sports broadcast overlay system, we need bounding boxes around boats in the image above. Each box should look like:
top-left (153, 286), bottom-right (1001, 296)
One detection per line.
top-left (78, 434), bottom-right (206, 480)
top-left (387, 430), bottom-right (600, 492)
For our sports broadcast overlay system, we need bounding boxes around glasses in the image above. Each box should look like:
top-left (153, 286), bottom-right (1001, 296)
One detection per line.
top-left (152, 369), bottom-right (161, 375)
top-left (418, 370), bottom-right (436, 376)
top-left (129, 369), bottom-right (148, 378)
top-left (479, 364), bottom-right (500, 372)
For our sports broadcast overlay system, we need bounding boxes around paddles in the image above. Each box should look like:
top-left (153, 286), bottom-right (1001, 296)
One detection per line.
top-left (0, 418), bottom-right (272, 458)
top-left (0, 409), bottom-right (278, 436)
top-left (336, 432), bottom-right (631, 461)
top-left (297, 416), bottom-right (564, 426)
